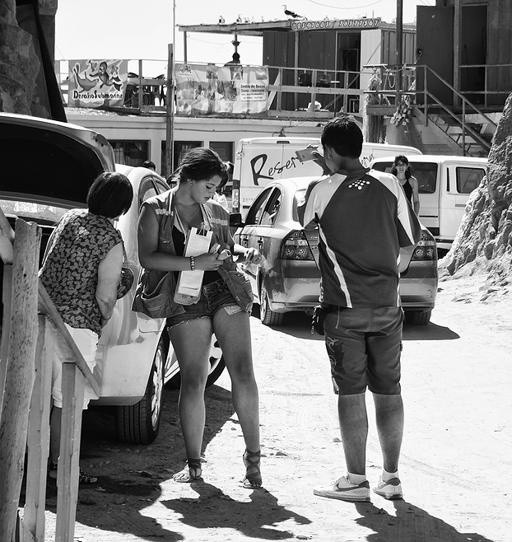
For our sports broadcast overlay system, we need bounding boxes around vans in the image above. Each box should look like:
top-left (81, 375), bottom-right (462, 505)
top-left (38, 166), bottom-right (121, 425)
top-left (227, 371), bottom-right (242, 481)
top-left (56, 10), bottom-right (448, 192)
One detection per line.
top-left (376, 155), bottom-right (492, 258)
top-left (235, 135), bottom-right (425, 222)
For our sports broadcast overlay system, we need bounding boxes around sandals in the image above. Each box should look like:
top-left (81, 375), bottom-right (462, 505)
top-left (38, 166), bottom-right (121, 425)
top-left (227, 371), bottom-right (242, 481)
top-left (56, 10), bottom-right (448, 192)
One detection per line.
top-left (241, 449), bottom-right (262, 488)
top-left (48, 461), bottom-right (99, 486)
top-left (172, 458), bottom-right (203, 483)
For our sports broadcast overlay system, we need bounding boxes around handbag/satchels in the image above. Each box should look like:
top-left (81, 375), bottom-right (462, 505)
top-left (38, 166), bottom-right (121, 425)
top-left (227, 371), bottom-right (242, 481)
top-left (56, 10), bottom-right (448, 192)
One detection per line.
top-left (117, 267), bottom-right (134, 299)
top-left (313, 305), bottom-right (326, 335)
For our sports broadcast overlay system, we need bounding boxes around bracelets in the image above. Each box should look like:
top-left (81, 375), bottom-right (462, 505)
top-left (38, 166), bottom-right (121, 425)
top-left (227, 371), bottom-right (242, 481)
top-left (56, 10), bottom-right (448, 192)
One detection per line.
top-left (189, 255), bottom-right (196, 271)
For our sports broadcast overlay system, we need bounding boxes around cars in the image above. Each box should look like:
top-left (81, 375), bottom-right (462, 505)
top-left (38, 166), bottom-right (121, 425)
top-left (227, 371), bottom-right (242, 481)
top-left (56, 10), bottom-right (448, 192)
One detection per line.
top-left (232, 176), bottom-right (438, 324)
top-left (0, 110), bottom-right (236, 441)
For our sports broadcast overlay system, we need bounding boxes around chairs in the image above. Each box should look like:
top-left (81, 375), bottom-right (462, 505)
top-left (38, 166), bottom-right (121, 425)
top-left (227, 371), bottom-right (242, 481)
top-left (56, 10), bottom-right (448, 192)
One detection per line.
top-left (124, 72), bottom-right (166, 107)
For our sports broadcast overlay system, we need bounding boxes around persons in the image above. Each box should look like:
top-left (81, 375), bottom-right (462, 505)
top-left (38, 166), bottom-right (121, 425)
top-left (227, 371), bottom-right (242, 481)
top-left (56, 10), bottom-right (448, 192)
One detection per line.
top-left (39, 171), bottom-right (133, 486)
top-left (131, 147), bottom-right (262, 490)
top-left (141, 160), bottom-right (156, 171)
top-left (223, 51), bottom-right (243, 64)
top-left (392, 155), bottom-right (421, 220)
top-left (298, 114), bottom-right (421, 504)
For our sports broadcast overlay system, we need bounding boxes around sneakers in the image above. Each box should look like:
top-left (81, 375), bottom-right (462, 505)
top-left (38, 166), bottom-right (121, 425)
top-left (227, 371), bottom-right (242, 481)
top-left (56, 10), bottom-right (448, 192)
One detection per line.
top-left (314, 476), bottom-right (370, 502)
top-left (373, 478), bottom-right (403, 500)
